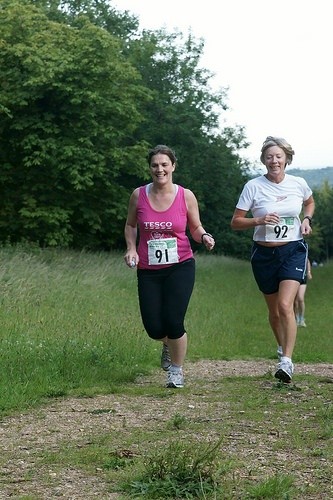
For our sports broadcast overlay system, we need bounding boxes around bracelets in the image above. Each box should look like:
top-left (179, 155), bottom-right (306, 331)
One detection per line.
top-left (201, 233), bottom-right (213, 244)
top-left (303, 216), bottom-right (312, 225)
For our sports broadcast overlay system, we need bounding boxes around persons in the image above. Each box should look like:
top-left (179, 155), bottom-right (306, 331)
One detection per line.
top-left (230, 136), bottom-right (315, 383)
top-left (124, 144), bottom-right (215, 389)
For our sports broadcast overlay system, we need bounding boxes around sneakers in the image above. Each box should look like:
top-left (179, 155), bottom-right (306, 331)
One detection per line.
top-left (276, 350), bottom-right (293, 382)
top-left (166, 367), bottom-right (184, 388)
top-left (296, 319), bottom-right (307, 327)
top-left (161, 343), bottom-right (171, 370)
